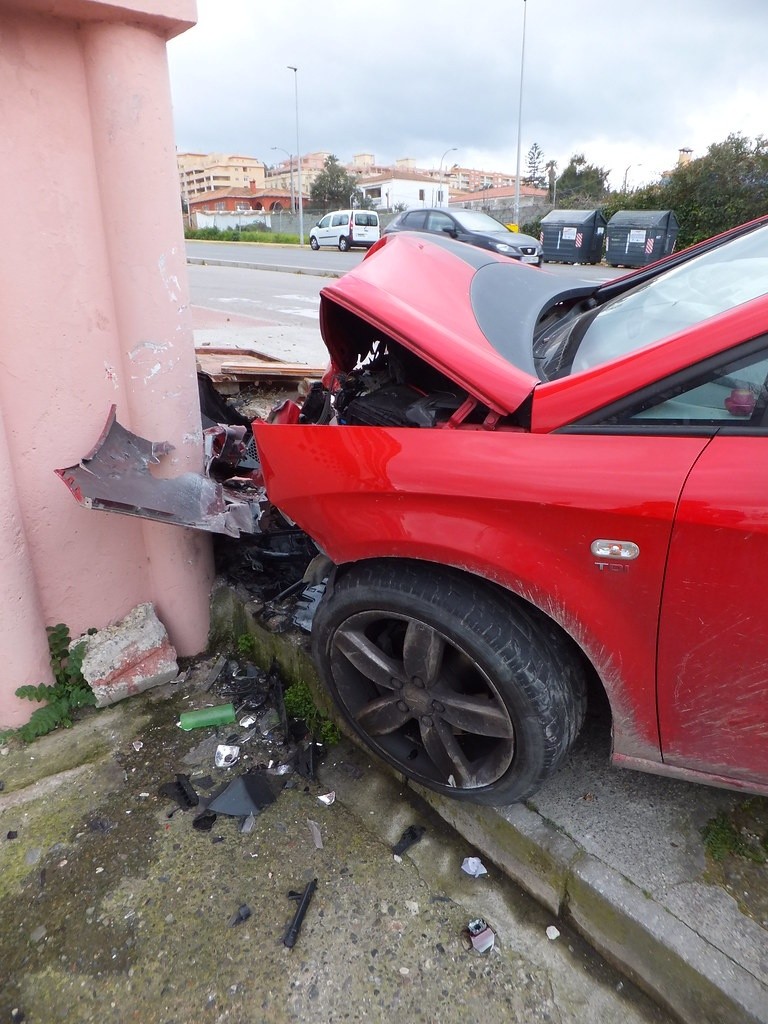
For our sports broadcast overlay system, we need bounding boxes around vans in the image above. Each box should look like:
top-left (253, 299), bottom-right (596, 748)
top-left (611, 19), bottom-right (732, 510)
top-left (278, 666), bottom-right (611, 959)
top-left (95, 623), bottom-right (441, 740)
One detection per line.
top-left (308, 210), bottom-right (381, 252)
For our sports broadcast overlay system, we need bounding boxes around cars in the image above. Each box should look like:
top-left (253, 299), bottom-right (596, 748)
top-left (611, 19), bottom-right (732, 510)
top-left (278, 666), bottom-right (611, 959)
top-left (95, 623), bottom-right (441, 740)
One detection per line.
top-left (209, 210), bottom-right (768, 802)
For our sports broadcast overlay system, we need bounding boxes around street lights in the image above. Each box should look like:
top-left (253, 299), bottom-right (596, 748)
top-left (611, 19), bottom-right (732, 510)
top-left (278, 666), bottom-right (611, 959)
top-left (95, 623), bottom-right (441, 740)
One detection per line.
top-left (271, 147), bottom-right (295, 215)
top-left (624, 163), bottom-right (642, 202)
top-left (286, 67), bottom-right (304, 246)
top-left (183, 200), bottom-right (191, 227)
top-left (349, 190), bottom-right (358, 210)
top-left (439, 148), bottom-right (458, 208)
top-left (554, 173), bottom-right (570, 207)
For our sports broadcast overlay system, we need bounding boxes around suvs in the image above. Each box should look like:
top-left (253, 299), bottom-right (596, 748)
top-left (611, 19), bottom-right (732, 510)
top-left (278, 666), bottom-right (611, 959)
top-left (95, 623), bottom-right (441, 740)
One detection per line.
top-left (381, 208), bottom-right (543, 267)
